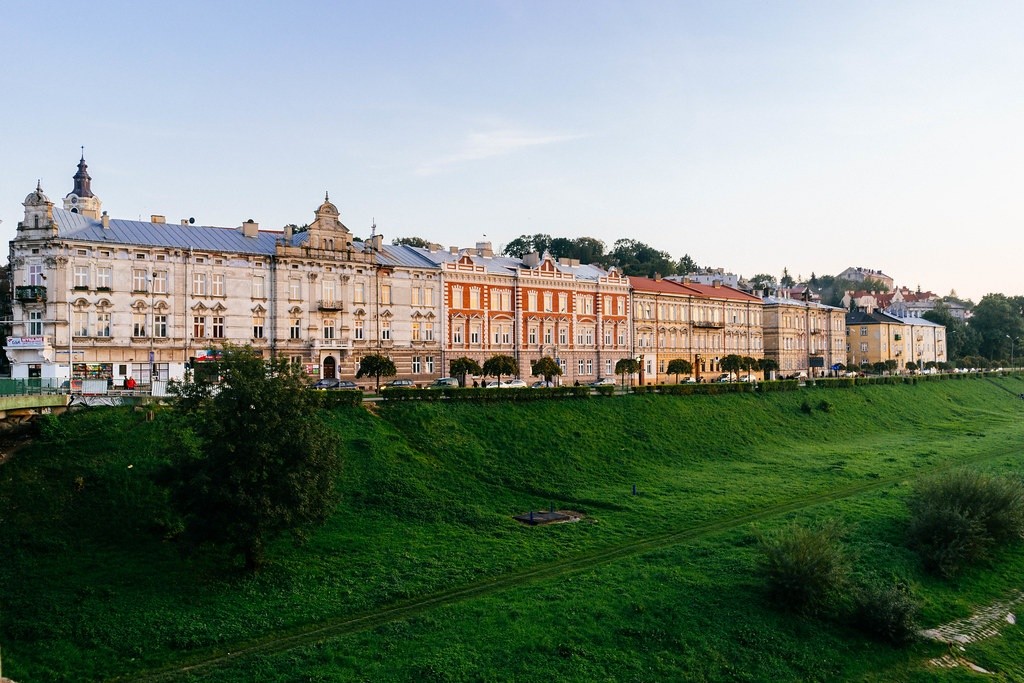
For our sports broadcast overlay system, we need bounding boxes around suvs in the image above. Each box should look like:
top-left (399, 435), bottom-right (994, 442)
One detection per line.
top-left (309, 377), bottom-right (341, 390)
top-left (431, 377), bottom-right (459, 389)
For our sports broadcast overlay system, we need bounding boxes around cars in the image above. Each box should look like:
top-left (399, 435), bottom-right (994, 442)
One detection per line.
top-left (917, 366), bottom-right (968, 376)
top-left (843, 369), bottom-right (859, 378)
top-left (511, 379), bottom-right (529, 390)
top-left (486, 381), bottom-right (510, 388)
top-left (531, 380), bottom-right (554, 389)
top-left (338, 380), bottom-right (359, 390)
top-left (681, 377), bottom-right (697, 385)
top-left (385, 378), bottom-right (416, 389)
top-left (60, 379), bottom-right (81, 393)
top-left (739, 374), bottom-right (756, 382)
top-left (592, 377), bottom-right (617, 387)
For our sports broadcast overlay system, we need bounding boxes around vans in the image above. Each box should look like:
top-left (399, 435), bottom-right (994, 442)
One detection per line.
top-left (716, 371), bottom-right (737, 383)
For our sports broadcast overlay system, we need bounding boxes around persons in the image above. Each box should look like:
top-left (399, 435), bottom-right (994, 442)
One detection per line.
top-left (480, 378), bottom-right (486, 387)
top-left (169, 377), bottom-right (175, 382)
top-left (574, 380), bottom-right (580, 386)
top-left (697, 376), bottom-right (707, 383)
top-left (472, 379), bottom-right (479, 388)
top-left (123, 376), bottom-right (136, 390)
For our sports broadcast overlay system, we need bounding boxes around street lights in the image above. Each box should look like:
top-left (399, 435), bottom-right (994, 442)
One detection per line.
top-left (1006, 334), bottom-right (1020, 371)
top-left (374, 264), bottom-right (384, 394)
